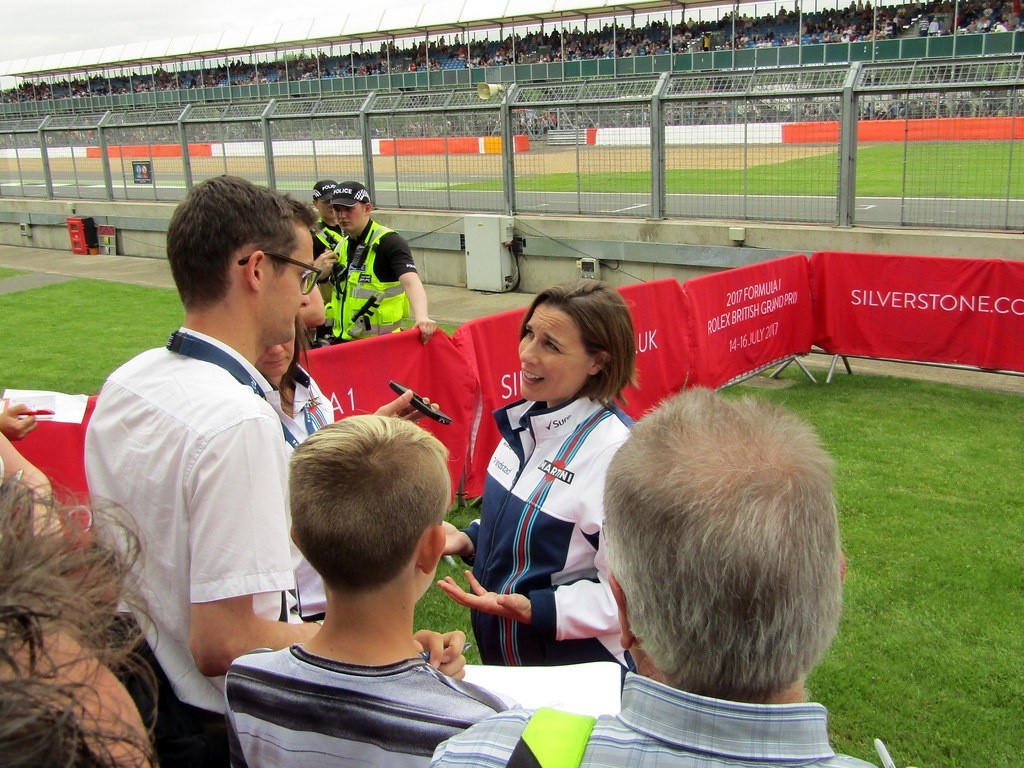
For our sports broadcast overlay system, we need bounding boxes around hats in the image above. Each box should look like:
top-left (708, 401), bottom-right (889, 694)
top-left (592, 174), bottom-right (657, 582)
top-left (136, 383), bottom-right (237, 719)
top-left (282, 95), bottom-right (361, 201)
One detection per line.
top-left (312, 179), bottom-right (337, 201)
top-left (329, 181), bottom-right (371, 207)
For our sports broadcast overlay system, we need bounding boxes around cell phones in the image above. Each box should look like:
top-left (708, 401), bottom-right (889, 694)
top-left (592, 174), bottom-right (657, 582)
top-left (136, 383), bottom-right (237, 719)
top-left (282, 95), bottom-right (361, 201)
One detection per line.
top-left (388, 381), bottom-right (452, 425)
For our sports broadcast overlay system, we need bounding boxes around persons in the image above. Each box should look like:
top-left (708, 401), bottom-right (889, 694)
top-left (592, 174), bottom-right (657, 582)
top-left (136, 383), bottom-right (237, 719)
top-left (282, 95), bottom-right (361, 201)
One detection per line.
top-left (223, 412), bottom-right (508, 767)
top-left (515, 28), bottom-right (562, 64)
top-left (615, 18), bottom-right (671, 57)
top-left (0, 40), bottom-right (387, 149)
top-left (802, 0), bottom-right (873, 44)
top-left (436, 280), bottom-right (642, 670)
top-left (564, 23), bottom-right (614, 61)
top-left (432, 384), bottom-right (881, 766)
top-left (85, 172), bottom-right (438, 768)
top-left (734, 6), bottom-right (799, 49)
top-left (389, 27), bottom-right (513, 73)
top-left (860, 93), bottom-right (1024, 119)
top-left (876, 0), bottom-right (956, 41)
top-left (392, 96), bottom-right (841, 134)
top-left (255, 315), bottom-right (335, 622)
top-left (957, 0), bottom-right (1024, 34)
top-left (672, 10), bottom-right (741, 53)
top-left (0, 402), bottom-right (156, 767)
top-left (309, 177), bottom-right (436, 345)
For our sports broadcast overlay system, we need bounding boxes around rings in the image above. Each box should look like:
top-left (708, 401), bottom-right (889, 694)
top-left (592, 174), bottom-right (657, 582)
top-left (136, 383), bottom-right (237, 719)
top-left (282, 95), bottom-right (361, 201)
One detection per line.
top-left (426, 335), bottom-right (428, 337)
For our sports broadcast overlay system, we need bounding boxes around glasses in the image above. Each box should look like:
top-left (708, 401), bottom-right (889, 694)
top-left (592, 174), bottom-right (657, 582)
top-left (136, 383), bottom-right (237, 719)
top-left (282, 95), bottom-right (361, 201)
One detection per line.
top-left (237, 251), bottom-right (322, 295)
